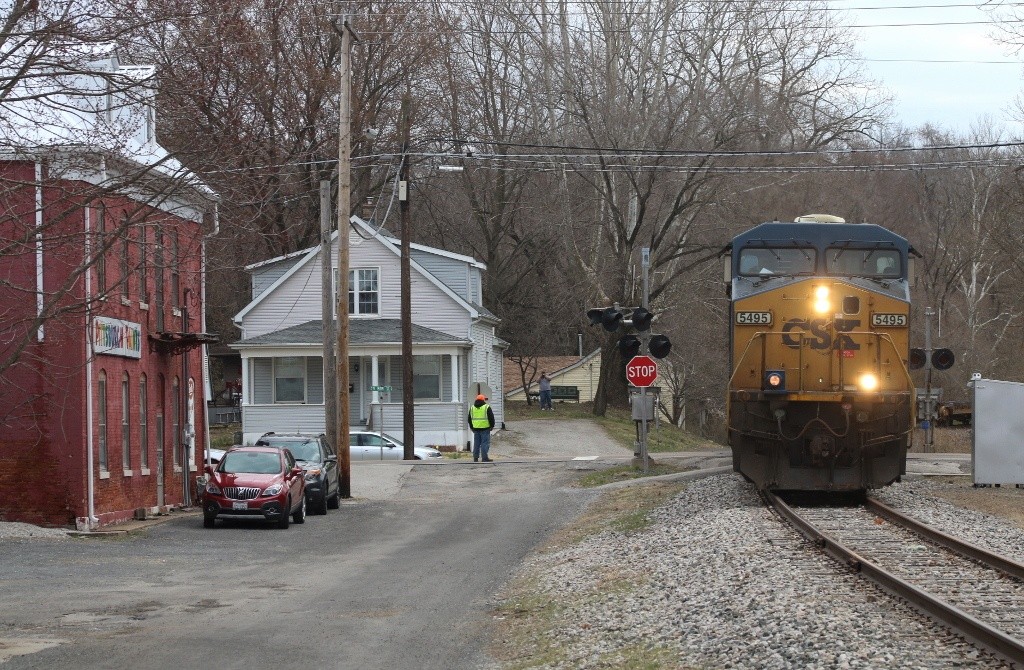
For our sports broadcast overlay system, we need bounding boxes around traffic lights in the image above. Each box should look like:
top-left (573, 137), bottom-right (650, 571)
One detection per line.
top-left (602, 308), bottom-right (623, 332)
top-left (911, 348), bottom-right (927, 369)
top-left (931, 348), bottom-right (955, 369)
top-left (649, 334), bottom-right (672, 359)
top-left (632, 308), bottom-right (653, 331)
top-left (587, 309), bottom-right (603, 327)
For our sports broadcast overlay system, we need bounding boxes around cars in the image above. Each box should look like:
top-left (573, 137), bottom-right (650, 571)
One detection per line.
top-left (204, 448), bottom-right (226, 483)
top-left (202, 446), bottom-right (307, 530)
top-left (349, 431), bottom-right (444, 461)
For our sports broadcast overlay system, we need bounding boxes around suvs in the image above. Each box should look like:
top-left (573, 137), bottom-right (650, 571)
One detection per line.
top-left (255, 431), bottom-right (341, 515)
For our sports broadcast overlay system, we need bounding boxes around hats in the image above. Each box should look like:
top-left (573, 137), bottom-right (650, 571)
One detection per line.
top-left (476, 395), bottom-right (484, 401)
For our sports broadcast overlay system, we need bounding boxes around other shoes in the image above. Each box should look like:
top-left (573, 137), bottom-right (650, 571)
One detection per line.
top-left (474, 458), bottom-right (478, 461)
top-left (542, 408), bottom-right (545, 410)
top-left (483, 458), bottom-right (493, 462)
top-left (548, 408), bottom-right (555, 411)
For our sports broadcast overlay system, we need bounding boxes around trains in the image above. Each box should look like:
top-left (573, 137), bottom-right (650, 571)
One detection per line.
top-left (724, 214), bottom-right (917, 496)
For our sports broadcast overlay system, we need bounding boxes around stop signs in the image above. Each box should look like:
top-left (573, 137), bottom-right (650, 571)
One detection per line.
top-left (626, 356), bottom-right (657, 388)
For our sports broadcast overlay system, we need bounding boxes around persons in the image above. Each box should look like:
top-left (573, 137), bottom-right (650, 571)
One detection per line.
top-left (468, 395), bottom-right (495, 462)
top-left (537, 371), bottom-right (555, 411)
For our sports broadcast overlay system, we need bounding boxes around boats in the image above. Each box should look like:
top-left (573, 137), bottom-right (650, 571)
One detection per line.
top-left (619, 335), bottom-right (642, 357)
